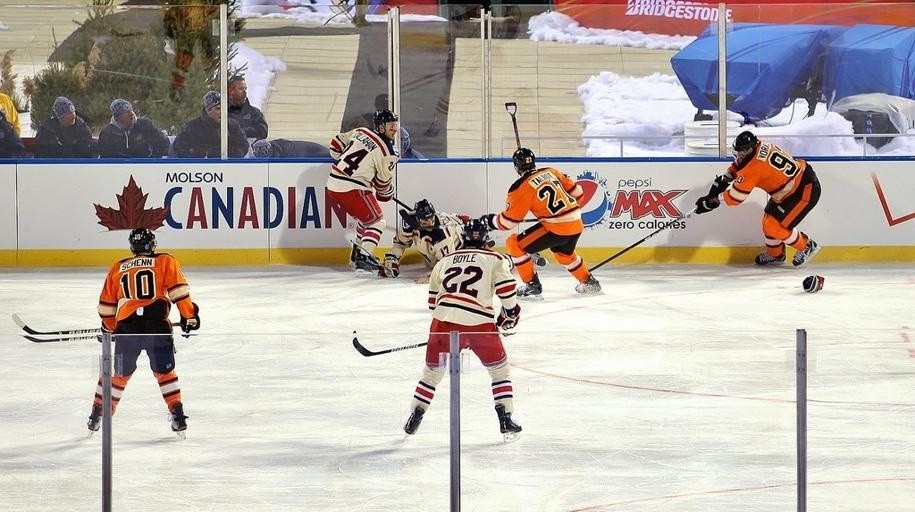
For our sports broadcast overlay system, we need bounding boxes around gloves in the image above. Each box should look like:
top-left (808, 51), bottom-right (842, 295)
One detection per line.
top-left (496, 305), bottom-right (520, 329)
top-left (98, 322), bottom-right (116, 343)
top-left (376, 184), bottom-right (395, 202)
top-left (181, 302), bottom-right (201, 332)
top-left (480, 214), bottom-right (498, 232)
top-left (709, 175), bottom-right (730, 197)
top-left (378, 253), bottom-right (402, 278)
top-left (694, 196), bottom-right (720, 214)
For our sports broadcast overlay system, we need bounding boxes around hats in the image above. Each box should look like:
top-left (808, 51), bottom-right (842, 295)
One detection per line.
top-left (110, 98), bottom-right (133, 118)
top-left (53, 96), bottom-right (76, 119)
top-left (252, 139), bottom-right (274, 158)
top-left (203, 91), bottom-right (221, 111)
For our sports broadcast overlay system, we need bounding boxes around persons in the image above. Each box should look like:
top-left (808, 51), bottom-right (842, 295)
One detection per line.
top-left (489, 149), bottom-right (599, 294)
top-left (326, 111), bottom-right (398, 269)
top-left (98, 99), bottom-right (170, 159)
top-left (404, 220), bottom-right (521, 434)
top-left (169, 78), bottom-right (331, 158)
top-left (340, 94), bottom-right (425, 159)
top-left (1, 93), bottom-right (34, 158)
top-left (34, 96), bottom-right (93, 158)
top-left (163, 0), bottom-right (223, 99)
top-left (696, 131), bottom-right (821, 269)
top-left (87, 227), bottom-right (201, 431)
top-left (379, 198), bottom-right (474, 283)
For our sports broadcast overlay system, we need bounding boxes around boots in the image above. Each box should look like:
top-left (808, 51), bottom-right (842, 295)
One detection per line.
top-left (425, 122), bottom-right (440, 137)
top-left (171, 403), bottom-right (189, 431)
top-left (403, 405), bottom-right (425, 434)
top-left (495, 404), bottom-right (522, 433)
top-left (754, 242), bottom-right (786, 264)
top-left (88, 406), bottom-right (101, 432)
top-left (516, 271), bottom-right (542, 296)
top-left (351, 244), bottom-right (360, 261)
top-left (575, 273), bottom-right (601, 293)
top-left (354, 247), bottom-right (380, 270)
top-left (791, 232), bottom-right (817, 265)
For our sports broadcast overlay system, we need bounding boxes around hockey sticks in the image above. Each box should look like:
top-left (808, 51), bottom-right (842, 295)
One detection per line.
top-left (11, 314), bottom-right (181, 335)
top-left (23, 335), bottom-right (97, 343)
top-left (352, 323), bottom-right (498, 356)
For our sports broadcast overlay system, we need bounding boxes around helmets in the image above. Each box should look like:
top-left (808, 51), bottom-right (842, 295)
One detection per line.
top-left (128, 227), bottom-right (158, 255)
top-left (459, 218), bottom-right (489, 248)
top-left (414, 199), bottom-right (437, 232)
top-left (731, 130), bottom-right (758, 164)
top-left (374, 108), bottom-right (400, 142)
top-left (374, 94), bottom-right (388, 112)
top-left (512, 148), bottom-right (536, 175)
